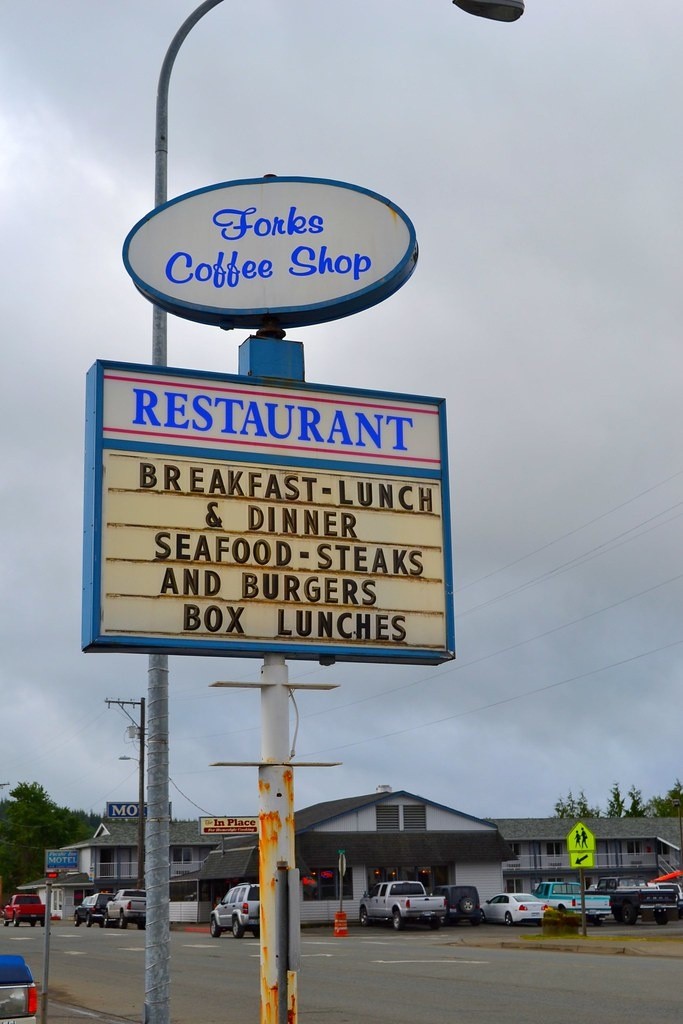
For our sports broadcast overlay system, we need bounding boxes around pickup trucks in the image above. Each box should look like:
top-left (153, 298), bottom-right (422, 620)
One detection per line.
top-left (105, 889), bottom-right (146, 930)
top-left (359, 881), bottom-right (448, 932)
top-left (533, 881), bottom-right (612, 927)
top-left (584, 877), bottom-right (679, 925)
top-left (73, 893), bottom-right (117, 928)
top-left (2, 894), bottom-right (46, 927)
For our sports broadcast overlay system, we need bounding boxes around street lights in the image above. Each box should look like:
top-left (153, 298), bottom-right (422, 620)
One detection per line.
top-left (119, 755), bottom-right (145, 888)
top-left (143, 0), bottom-right (528, 1024)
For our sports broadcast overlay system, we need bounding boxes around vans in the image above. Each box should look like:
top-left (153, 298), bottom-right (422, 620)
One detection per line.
top-left (632, 882), bottom-right (683, 920)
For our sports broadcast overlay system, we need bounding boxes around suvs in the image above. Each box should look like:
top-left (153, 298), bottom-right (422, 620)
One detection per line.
top-left (210, 882), bottom-right (260, 939)
top-left (428, 885), bottom-right (481, 927)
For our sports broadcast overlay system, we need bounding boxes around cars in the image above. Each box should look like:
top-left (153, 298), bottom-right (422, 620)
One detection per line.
top-left (479, 892), bottom-right (549, 927)
top-left (1, 951), bottom-right (38, 1024)
